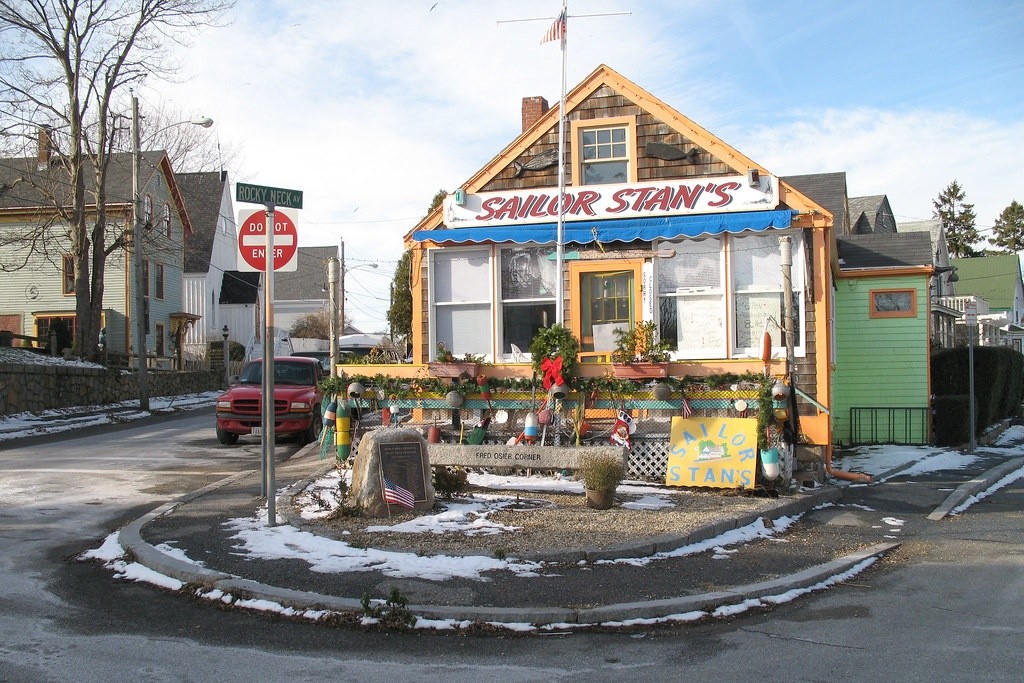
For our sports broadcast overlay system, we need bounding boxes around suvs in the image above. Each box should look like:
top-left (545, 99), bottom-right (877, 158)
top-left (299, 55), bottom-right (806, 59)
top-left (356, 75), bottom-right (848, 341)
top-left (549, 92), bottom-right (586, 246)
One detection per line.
top-left (215, 356), bottom-right (330, 445)
top-left (289, 351), bottom-right (355, 377)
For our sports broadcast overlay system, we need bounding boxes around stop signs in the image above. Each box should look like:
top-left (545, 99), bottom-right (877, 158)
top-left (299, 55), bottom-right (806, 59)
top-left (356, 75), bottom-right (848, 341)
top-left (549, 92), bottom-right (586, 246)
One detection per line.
top-left (238, 208), bottom-right (298, 273)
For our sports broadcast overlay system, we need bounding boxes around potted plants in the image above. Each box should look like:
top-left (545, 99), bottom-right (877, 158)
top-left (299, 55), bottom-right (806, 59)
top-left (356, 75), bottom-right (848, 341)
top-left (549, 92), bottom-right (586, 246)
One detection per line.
top-left (610, 319), bottom-right (678, 378)
top-left (426, 343), bottom-right (494, 379)
top-left (573, 449), bottom-right (625, 510)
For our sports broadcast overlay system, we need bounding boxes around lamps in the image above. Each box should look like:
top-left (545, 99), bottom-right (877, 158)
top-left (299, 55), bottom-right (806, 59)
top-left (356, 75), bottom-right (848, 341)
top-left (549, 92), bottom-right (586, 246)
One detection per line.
top-left (934, 266), bottom-right (959, 282)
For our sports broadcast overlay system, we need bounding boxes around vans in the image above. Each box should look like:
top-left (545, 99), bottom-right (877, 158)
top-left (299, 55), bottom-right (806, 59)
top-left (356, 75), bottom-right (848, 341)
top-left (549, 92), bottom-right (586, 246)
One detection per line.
top-left (338, 333), bottom-right (390, 359)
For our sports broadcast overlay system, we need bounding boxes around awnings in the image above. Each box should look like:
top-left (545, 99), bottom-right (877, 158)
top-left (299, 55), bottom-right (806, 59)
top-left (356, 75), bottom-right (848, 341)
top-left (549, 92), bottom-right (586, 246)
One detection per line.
top-left (412, 211), bottom-right (804, 244)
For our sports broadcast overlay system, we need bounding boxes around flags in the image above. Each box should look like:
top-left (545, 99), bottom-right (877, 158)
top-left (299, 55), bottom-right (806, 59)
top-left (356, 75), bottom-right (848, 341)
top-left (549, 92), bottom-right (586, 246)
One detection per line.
top-left (540, 9), bottom-right (566, 43)
top-left (385, 480), bottom-right (416, 510)
top-left (682, 398), bottom-right (691, 420)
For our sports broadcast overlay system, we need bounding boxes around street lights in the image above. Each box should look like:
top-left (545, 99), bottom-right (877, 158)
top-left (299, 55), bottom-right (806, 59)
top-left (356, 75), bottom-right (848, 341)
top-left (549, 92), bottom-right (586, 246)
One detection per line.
top-left (341, 241), bottom-right (380, 337)
top-left (132, 96), bottom-right (213, 411)
